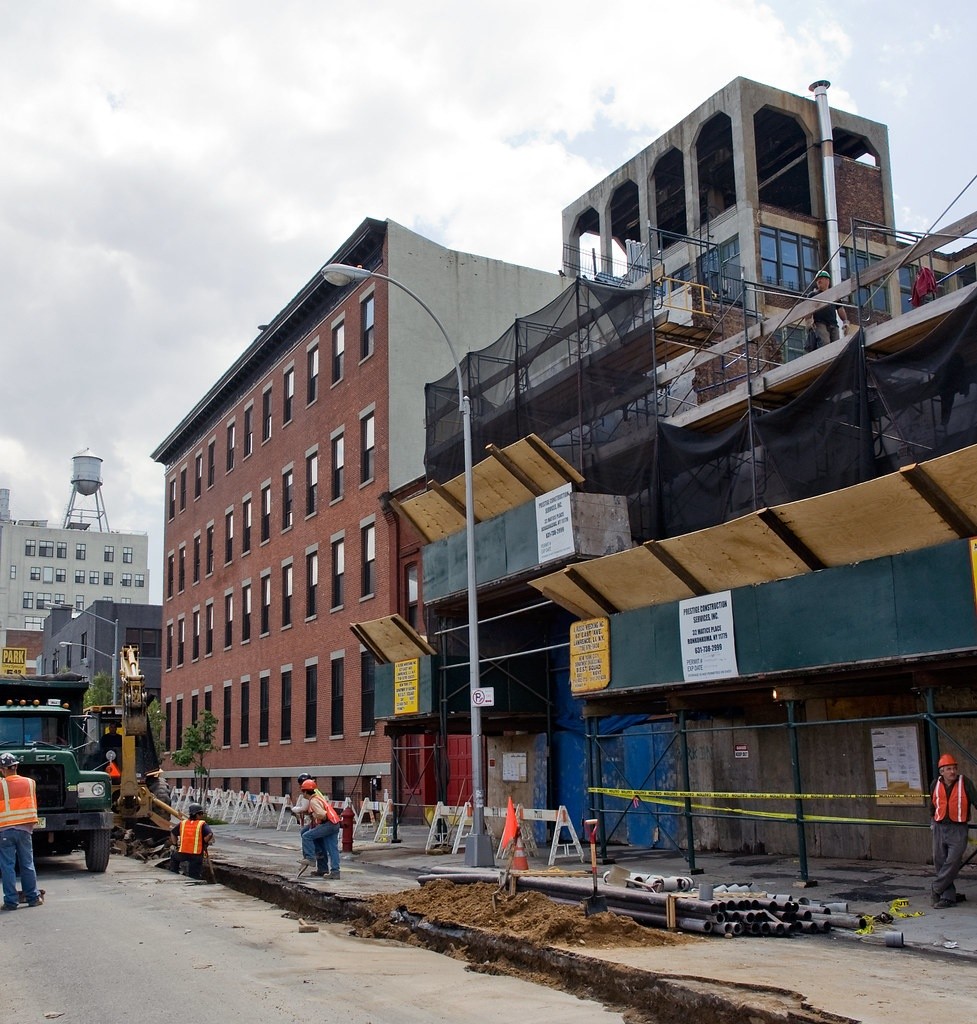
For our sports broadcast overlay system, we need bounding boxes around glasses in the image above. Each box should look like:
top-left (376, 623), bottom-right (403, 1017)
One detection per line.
top-left (198, 811), bottom-right (203, 815)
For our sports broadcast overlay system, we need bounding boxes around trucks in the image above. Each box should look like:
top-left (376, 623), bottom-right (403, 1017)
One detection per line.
top-left (0, 671), bottom-right (110, 872)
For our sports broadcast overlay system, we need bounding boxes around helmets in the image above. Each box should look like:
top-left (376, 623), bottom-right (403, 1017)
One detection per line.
top-left (816, 271), bottom-right (831, 279)
top-left (188, 803), bottom-right (203, 814)
top-left (301, 779), bottom-right (319, 790)
top-left (298, 773), bottom-right (312, 783)
top-left (938, 753), bottom-right (959, 768)
top-left (0, 752), bottom-right (20, 768)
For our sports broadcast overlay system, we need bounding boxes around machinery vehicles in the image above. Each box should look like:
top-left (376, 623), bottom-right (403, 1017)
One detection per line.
top-left (79, 642), bottom-right (189, 832)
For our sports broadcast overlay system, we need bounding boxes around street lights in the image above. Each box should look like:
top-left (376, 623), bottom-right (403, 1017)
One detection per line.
top-left (321, 264), bottom-right (495, 867)
top-left (43, 601), bottom-right (119, 705)
top-left (58, 639), bottom-right (115, 688)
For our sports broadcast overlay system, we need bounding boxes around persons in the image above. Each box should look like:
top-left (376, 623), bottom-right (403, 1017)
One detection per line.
top-left (0, 753), bottom-right (42, 910)
top-left (805, 271), bottom-right (850, 347)
top-left (291, 773), bottom-right (329, 876)
top-left (298, 780), bottom-right (341, 879)
top-left (99, 723), bottom-right (122, 748)
top-left (168, 803), bottom-right (213, 878)
top-left (930, 753), bottom-right (977, 909)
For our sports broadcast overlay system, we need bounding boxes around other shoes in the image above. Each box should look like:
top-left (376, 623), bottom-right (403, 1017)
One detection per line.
top-left (296, 858), bottom-right (316, 867)
top-left (933, 898), bottom-right (957, 909)
top-left (929, 880), bottom-right (941, 907)
top-left (324, 872), bottom-right (340, 880)
top-left (311, 871), bottom-right (329, 876)
top-left (0, 903), bottom-right (18, 911)
top-left (29, 896), bottom-right (44, 906)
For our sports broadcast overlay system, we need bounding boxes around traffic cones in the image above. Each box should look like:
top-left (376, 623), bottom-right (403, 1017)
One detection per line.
top-left (510, 832), bottom-right (529, 872)
